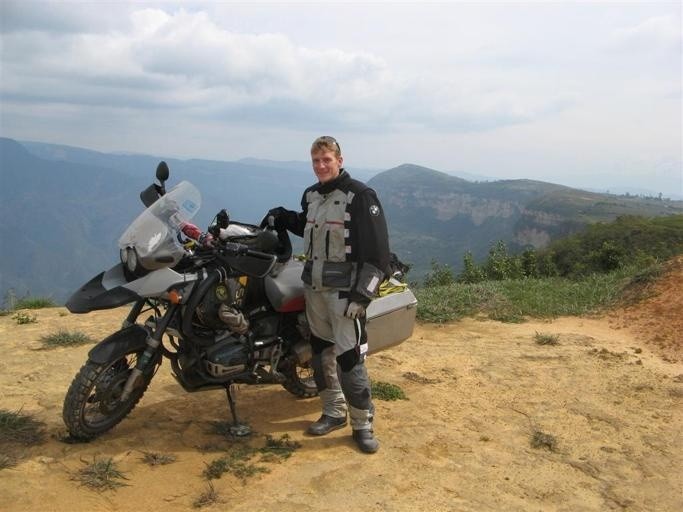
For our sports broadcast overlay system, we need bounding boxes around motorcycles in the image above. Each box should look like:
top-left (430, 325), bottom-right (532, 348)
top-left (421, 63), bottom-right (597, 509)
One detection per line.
top-left (61, 158), bottom-right (419, 443)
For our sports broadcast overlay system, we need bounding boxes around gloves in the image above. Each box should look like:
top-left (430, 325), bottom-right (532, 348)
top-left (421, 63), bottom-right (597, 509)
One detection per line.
top-left (343, 301), bottom-right (366, 320)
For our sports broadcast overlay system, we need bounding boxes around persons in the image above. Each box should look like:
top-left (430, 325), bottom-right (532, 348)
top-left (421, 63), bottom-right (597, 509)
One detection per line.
top-left (264, 136), bottom-right (392, 453)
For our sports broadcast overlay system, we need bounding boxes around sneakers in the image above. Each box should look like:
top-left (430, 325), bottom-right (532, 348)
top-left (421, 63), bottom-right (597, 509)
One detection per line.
top-left (308, 411), bottom-right (348, 435)
top-left (352, 426), bottom-right (378, 454)
